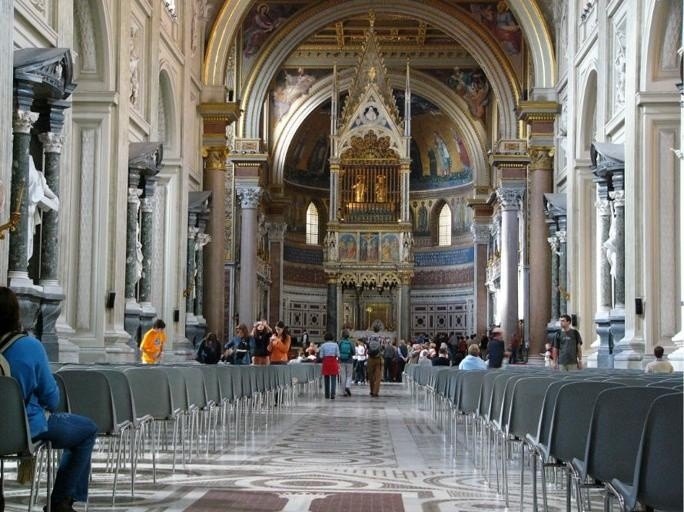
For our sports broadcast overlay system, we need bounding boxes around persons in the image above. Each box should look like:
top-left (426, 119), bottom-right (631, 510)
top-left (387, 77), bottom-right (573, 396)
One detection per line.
top-left (337, 329), bottom-right (356, 397)
top-left (365, 324), bottom-right (385, 397)
top-left (300, 314), bottom-right (584, 371)
top-left (139, 318), bottom-right (167, 365)
top-left (319, 332), bottom-right (341, 399)
top-left (644, 345), bottom-right (674, 374)
top-left (223, 323), bottom-right (257, 366)
top-left (197, 332), bottom-right (222, 364)
top-left (0, 285), bottom-right (97, 512)
top-left (249, 320), bottom-right (274, 366)
top-left (267, 320), bottom-right (291, 365)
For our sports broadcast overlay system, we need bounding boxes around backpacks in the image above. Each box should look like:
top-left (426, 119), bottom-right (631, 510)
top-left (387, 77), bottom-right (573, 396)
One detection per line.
top-left (0, 328), bottom-right (30, 377)
top-left (338, 338), bottom-right (352, 362)
top-left (367, 340), bottom-right (382, 357)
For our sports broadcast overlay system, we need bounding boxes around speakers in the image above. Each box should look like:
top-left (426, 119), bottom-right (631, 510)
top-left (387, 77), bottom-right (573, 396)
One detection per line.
top-left (106, 291), bottom-right (116, 309)
top-left (571, 313), bottom-right (577, 327)
top-left (172, 309), bottom-right (180, 323)
top-left (634, 297), bottom-right (644, 315)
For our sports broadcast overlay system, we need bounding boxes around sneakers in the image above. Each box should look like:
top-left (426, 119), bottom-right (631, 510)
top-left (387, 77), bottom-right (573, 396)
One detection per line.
top-left (43, 497), bottom-right (76, 512)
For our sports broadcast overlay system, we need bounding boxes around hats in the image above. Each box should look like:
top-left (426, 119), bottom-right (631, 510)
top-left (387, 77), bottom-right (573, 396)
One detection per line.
top-left (492, 328), bottom-right (505, 334)
